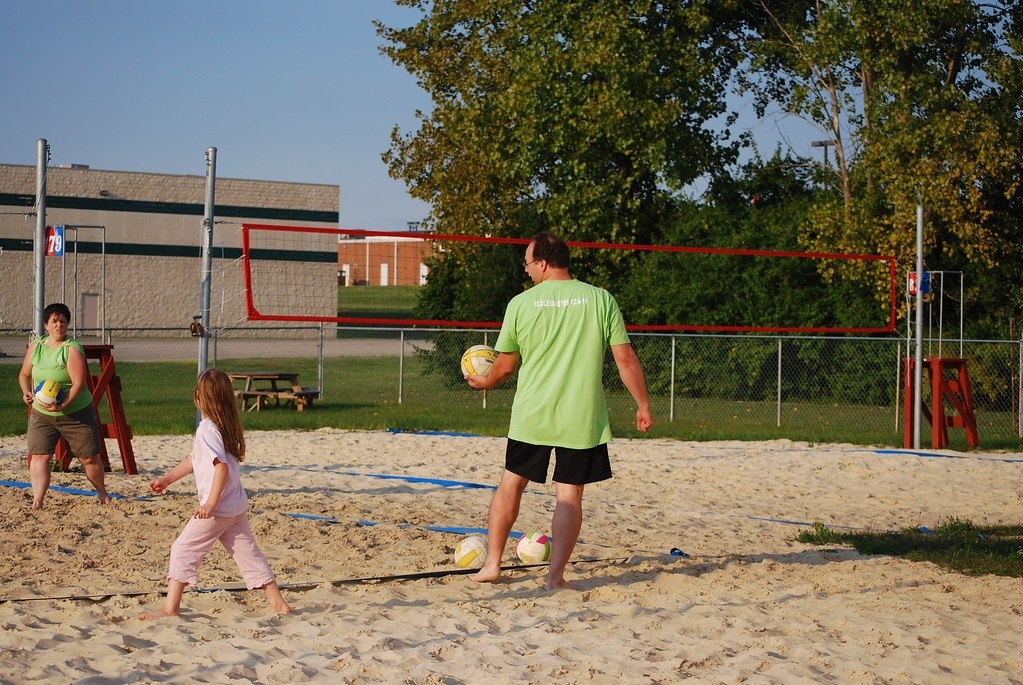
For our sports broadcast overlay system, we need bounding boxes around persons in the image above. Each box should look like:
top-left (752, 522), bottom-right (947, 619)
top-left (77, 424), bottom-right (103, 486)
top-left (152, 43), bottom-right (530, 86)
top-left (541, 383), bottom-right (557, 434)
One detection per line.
top-left (19, 303), bottom-right (111, 508)
top-left (137, 370), bottom-right (290, 620)
top-left (464, 232), bottom-right (653, 589)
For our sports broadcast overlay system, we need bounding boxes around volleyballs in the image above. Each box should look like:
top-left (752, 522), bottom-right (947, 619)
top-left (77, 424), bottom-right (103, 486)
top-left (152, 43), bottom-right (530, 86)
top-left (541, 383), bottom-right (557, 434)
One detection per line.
top-left (35, 379), bottom-right (64, 408)
top-left (454, 535), bottom-right (489, 569)
top-left (460, 344), bottom-right (499, 382)
top-left (515, 530), bottom-right (552, 564)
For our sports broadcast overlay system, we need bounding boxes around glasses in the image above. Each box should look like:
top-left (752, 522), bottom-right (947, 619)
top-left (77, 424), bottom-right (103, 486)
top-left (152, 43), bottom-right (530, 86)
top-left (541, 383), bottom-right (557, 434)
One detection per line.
top-left (522, 258), bottom-right (540, 269)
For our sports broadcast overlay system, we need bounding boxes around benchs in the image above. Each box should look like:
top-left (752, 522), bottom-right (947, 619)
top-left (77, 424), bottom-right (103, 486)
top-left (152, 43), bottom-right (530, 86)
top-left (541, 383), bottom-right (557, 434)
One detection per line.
top-left (250, 386), bottom-right (321, 409)
top-left (233, 389), bottom-right (310, 412)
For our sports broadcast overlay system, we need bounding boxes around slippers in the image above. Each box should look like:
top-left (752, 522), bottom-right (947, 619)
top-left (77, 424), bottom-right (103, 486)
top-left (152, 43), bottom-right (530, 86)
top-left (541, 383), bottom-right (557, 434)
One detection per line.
top-left (670, 548), bottom-right (690, 558)
top-left (918, 527), bottom-right (935, 535)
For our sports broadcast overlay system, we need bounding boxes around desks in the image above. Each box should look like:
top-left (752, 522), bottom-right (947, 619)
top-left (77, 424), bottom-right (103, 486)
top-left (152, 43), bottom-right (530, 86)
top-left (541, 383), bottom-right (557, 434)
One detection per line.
top-left (226, 371), bottom-right (303, 411)
